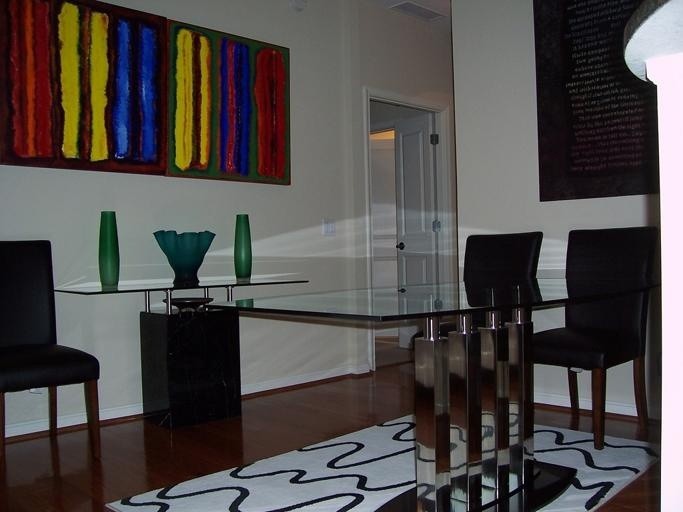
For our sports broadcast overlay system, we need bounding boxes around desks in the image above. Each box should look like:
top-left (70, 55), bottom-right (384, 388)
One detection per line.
top-left (54, 276), bottom-right (308, 429)
top-left (202, 276), bottom-right (660, 511)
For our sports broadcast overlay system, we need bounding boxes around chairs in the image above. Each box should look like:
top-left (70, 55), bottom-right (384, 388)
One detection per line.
top-left (0, 239), bottom-right (103, 485)
top-left (406, 232), bottom-right (545, 350)
top-left (521, 226), bottom-right (657, 452)
